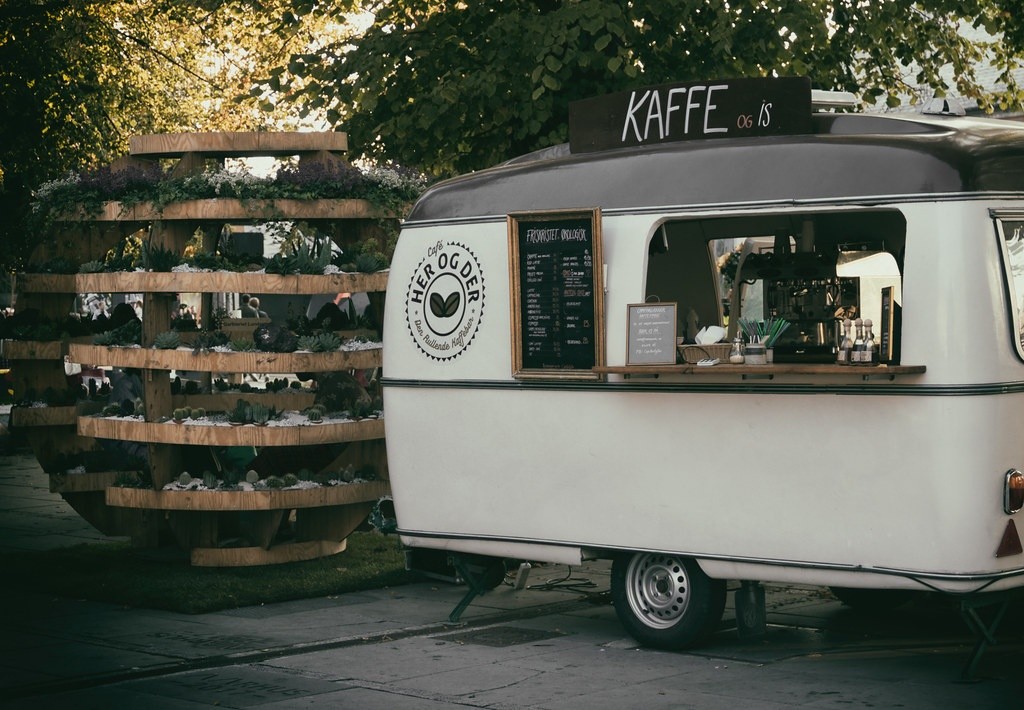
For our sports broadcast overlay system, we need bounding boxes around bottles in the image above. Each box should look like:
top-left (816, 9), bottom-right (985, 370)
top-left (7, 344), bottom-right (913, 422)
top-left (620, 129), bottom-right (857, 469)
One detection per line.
top-left (685, 306), bottom-right (699, 340)
top-left (730, 331), bottom-right (745, 364)
top-left (838, 318), bottom-right (879, 366)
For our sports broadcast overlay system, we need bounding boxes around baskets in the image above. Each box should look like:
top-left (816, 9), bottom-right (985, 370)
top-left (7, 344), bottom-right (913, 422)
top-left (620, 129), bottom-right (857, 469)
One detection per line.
top-left (679, 344), bottom-right (732, 364)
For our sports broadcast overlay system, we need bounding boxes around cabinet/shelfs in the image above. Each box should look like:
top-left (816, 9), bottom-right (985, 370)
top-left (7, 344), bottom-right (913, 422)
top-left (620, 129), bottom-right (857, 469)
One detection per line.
top-left (7, 130), bottom-right (429, 566)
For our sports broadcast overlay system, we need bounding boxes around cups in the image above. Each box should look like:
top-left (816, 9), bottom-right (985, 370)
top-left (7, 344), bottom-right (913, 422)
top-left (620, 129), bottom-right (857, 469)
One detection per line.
top-left (774, 229), bottom-right (792, 277)
top-left (799, 220), bottom-right (816, 252)
top-left (677, 337), bottom-right (684, 345)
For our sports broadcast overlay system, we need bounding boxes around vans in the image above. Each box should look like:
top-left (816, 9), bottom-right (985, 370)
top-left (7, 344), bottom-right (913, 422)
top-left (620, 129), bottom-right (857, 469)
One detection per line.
top-left (377, 75), bottom-right (1023, 651)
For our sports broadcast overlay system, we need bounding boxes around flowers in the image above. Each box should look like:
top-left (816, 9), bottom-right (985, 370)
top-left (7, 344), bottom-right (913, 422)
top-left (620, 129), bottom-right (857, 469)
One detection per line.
top-left (30, 155), bottom-right (428, 221)
